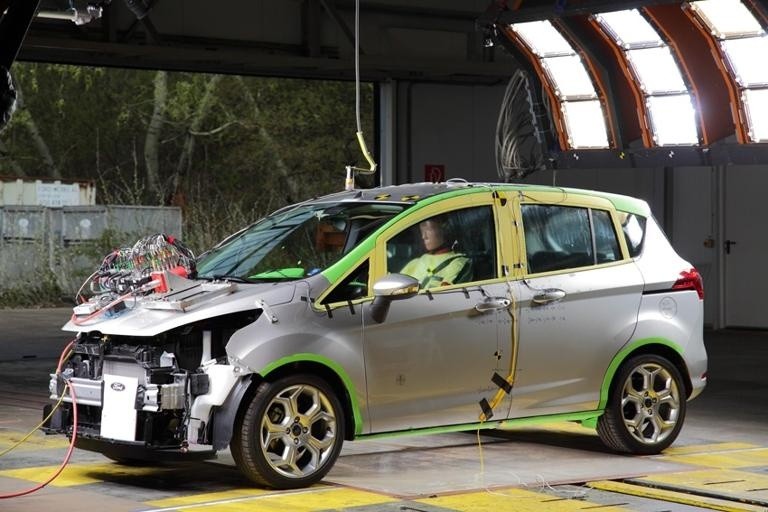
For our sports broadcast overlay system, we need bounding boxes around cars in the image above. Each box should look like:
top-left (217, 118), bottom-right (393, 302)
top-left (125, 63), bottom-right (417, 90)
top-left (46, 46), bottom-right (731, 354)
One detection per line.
top-left (42, 179), bottom-right (714, 494)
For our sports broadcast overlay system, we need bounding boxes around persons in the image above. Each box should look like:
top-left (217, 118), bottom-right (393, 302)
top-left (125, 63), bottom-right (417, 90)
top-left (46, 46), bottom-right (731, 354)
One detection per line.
top-left (398, 214), bottom-right (474, 292)
